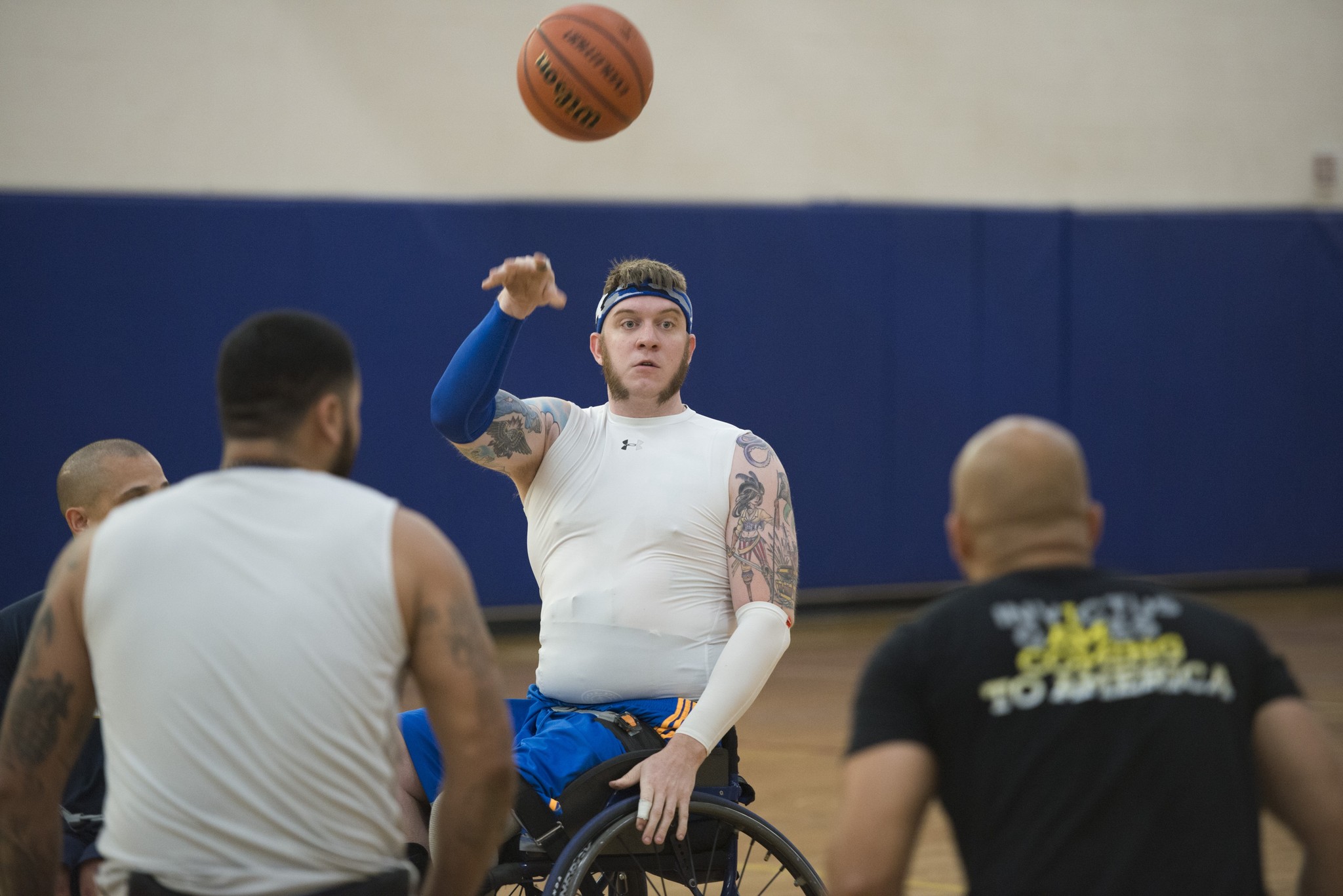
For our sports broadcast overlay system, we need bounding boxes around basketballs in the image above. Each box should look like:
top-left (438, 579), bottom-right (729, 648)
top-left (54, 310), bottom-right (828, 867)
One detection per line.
top-left (517, 4), bottom-right (653, 143)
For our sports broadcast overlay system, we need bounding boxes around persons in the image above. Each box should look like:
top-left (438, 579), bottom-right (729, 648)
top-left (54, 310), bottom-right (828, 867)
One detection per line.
top-left (0, 438), bottom-right (173, 896)
top-left (826, 411), bottom-right (1343, 896)
top-left (398, 251), bottom-right (799, 896)
top-left (0, 309), bottom-right (515, 896)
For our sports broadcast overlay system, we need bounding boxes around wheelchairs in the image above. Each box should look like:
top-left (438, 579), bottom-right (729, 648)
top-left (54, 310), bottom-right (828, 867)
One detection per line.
top-left (417, 745), bottom-right (830, 896)
